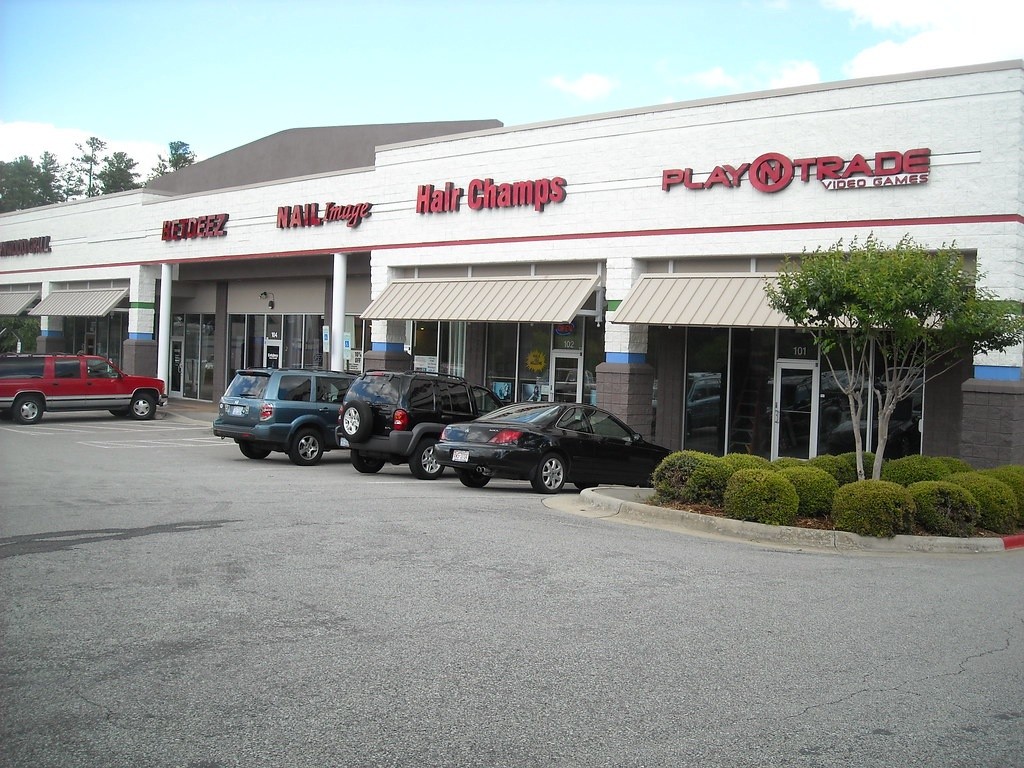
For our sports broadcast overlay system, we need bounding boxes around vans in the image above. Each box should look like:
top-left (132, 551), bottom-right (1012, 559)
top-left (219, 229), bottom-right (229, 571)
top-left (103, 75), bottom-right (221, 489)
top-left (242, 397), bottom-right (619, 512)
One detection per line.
top-left (536, 368), bottom-right (596, 402)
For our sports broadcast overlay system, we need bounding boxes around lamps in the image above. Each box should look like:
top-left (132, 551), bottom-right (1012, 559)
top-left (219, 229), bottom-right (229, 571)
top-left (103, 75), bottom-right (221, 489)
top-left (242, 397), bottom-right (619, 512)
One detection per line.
top-left (260, 292), bottom-right (275, 309)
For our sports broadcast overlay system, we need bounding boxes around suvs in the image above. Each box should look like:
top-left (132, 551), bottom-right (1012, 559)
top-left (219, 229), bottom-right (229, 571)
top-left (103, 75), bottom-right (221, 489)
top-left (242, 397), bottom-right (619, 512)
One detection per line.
top-left (0, 352), bottom-right (169, 424)
top-left (682, 373), bottom-right (723, 439)
top-left (762, 374), bottom-right (842, 452)
top-left (339, 369), bottom-right (504, 480)
top-left (212, 367), bottom-right (362, 466)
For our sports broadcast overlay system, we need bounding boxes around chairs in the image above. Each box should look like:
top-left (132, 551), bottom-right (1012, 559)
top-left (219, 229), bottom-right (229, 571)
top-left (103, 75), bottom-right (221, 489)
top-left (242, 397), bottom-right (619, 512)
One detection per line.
top-left (572, 419), bottom-right (584, 432)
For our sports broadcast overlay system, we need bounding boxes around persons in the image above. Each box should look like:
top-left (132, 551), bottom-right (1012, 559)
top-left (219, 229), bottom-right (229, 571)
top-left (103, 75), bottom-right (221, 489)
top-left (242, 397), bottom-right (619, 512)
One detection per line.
top-left (528, 386), bottom-right (540, 402)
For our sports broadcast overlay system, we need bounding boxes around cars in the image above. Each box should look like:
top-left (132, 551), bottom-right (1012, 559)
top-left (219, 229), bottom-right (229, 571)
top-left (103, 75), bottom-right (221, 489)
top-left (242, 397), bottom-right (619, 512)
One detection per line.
top-left (433, 400), bottom-right (673, 493)
top-left (881, 367), bottom-right (923, 409)
